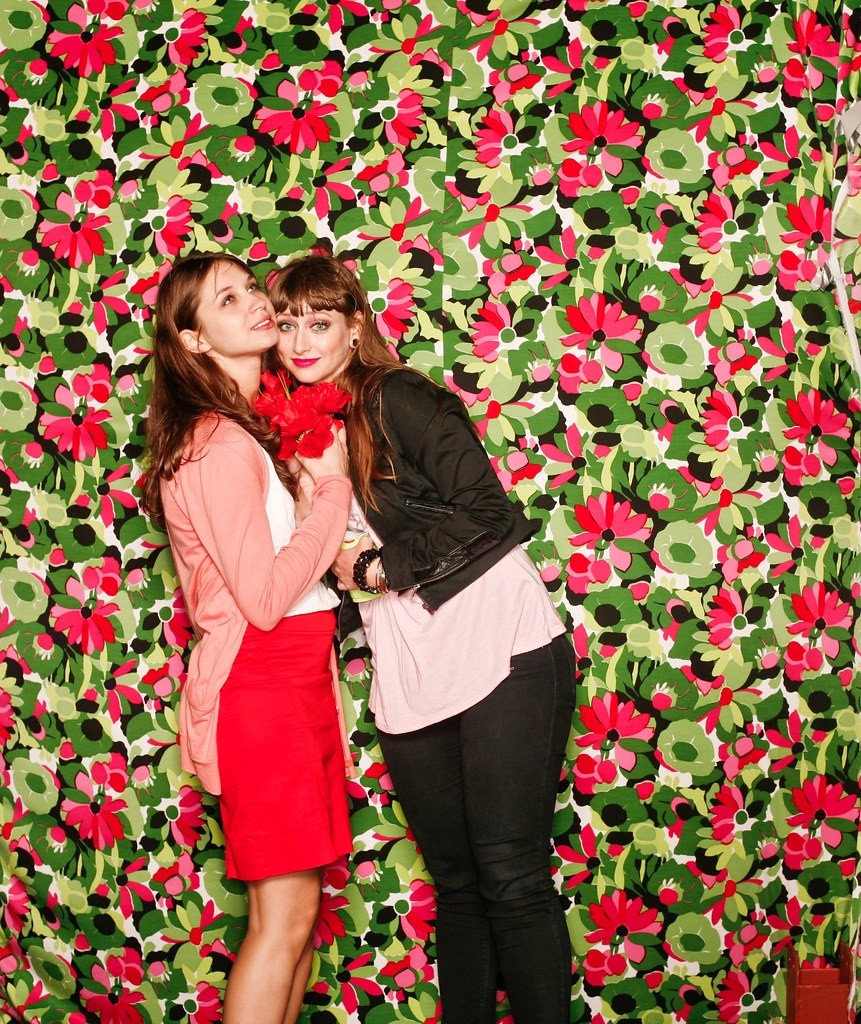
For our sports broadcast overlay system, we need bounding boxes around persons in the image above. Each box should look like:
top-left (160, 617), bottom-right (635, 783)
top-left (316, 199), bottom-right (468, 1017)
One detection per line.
top-left (137, 254), bottom-right (352, 1024)
top-left (260, 255), bottom-right (576, 1024)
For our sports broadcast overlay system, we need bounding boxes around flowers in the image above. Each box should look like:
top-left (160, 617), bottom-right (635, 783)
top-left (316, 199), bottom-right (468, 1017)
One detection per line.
top-left (252, 367), bottom-right (351, 459)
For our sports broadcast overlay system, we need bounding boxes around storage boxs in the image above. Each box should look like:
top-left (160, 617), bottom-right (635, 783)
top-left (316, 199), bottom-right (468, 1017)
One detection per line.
top-left (786, 942), bottom-right (852, 1024)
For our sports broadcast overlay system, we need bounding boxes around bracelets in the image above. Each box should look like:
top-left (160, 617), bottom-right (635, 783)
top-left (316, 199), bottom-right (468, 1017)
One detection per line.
top-left (353, 549), bottom-right (390, 594)
